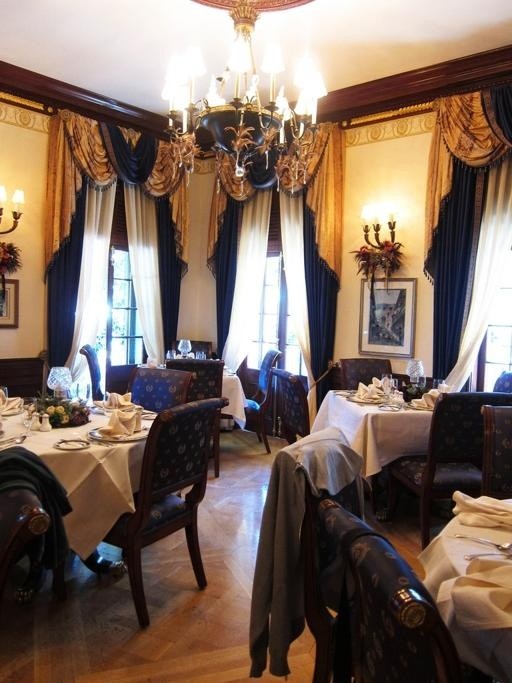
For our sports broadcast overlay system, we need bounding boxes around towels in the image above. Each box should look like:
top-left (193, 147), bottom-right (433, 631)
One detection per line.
top-left (411, 389), bottom-right (441, 412)
top-left (436, 558), bottom-right (512, 630)
top-left (352, 382), bottom-right (380, 403)
top-left (0, 418), bottom-right (8, 438)
top-left (452, 490), bottom-right (512, 527)
top-left (372, 376), bottom-right (392, 395)
top-left (98, 412), bottom-right (141, 440)
top-left (0, 390), bottom-right (25, 416)
top-left (94, 392), bottom-right (135, 413)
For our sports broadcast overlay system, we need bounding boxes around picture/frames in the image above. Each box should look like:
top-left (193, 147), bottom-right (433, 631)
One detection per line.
top-left (0, 278), bottom-right (20, 330)
top-left (358, 278), bottom-right (417, 358)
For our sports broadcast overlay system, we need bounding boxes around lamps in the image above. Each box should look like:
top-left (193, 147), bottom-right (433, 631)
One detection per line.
top-left (362, 203), bottom-right (371, 248)
top-left (169, 7), bottom-right (327, 196)
top-left (0, 183), bottom-right (26, 235)
top-left (372, 203), bottom-right (385, 248)
top-left (386, 203), bottom-right (400, 246)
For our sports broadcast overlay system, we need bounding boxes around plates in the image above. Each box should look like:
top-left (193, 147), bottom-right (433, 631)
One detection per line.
top-left (92, 401), bottom-right (133, 412)
top-left (378, 405), bottom-right (400, 411)
top-left (333, 390), bottom-right (351, 396)
top-left (88, 426), bottom-right (150, 442)
top-left (408, 402), bottom-right (434, 410)
top-left (1, 407), bottom-right (25, 416)
top-left (349, 396), bottom-right (387, 404)
top-left (55, 439), bottom-right (91, 450)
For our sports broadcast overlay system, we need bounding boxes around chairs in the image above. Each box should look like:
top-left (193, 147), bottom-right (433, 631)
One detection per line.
top-left (392, 393), bottom-right (512, 555)
top-left (492, 372), bottom-right (512, 394)
top-left (271, 367), bottom-right (311, 447)
top-left (0, 453), bottom-right (50, 578)
top-left (80, 345), bottom-right (106, 405)
top-left (165, 359), bottom-right (222, 478)
top-left (126, 367), bottom-right (191, 413)
top-left (340, 358), bottom-right (393, 392)
top-left (84, 397), bottom-right (220, 629)
top-left (221, 348), bottom-right (282, 453)
top-left (171, 340), bottom-right (213, 361)
top-left (281, 428), bottom-right (367, 683)
top-left (314, 499), bottom-right (453, 683)
top-left (480, 405), bottom-right (512, 500)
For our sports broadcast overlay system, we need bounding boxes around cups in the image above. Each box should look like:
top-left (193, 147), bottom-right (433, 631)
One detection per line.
top-left (22, 397), bottom-right (36, 419)
top-left (392, 379), bottom-right (398, 392)
top-left (406, 359), bottom-right (424, 383)
top-left (432, 379), bottom-right (445, 389)
top-left (198, 354), bottom-right (206, 360)
top-left (103, 393), bottom-right (119, 417)
top-left (178, 339), bottom-right (191, 357)
top-left (196, 351), bottom-right (204, 359)
top-left (77, 383), bottom-right (91, 404)
top-left (167, 350), bottom-right (176, 359)
top-left (381, 373), bottom-right (393, 395)
top-left (46, 367), bottom-right (74, 399)
top-left (23, 415), bottom-right (33, 437)
top-left (0, 386), bottom-right (8, 424)
top-left (147, 357), bottom-right (157, 368)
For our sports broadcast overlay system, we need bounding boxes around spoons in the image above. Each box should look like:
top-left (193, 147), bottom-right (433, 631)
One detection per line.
top-left (0, 436), bottom-right (27, 449)
top-left (455, 534), bottom-right (512, 551)
